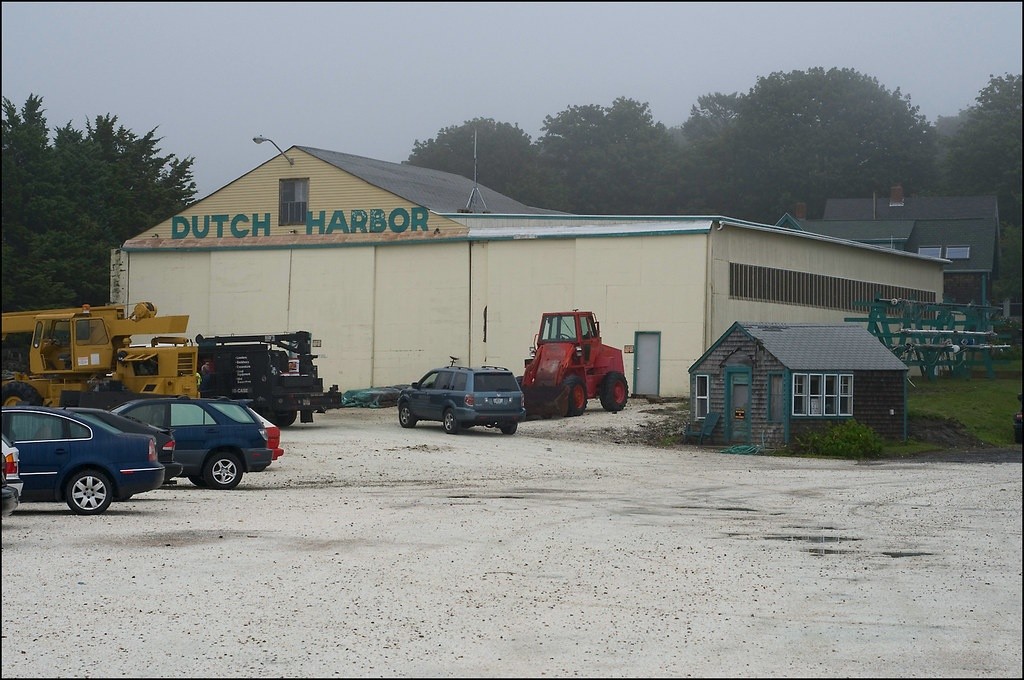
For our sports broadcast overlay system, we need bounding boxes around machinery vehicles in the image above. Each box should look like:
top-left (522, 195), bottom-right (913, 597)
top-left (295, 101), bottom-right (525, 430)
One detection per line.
top-left (2, 301), bottom-right (345, 430)
top-left (514, 310), bottom-right (630, 417)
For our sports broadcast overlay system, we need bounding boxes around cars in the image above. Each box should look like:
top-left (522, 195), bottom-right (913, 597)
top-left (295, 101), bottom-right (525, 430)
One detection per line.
top-left (0, 395), bottom-right (285, 516)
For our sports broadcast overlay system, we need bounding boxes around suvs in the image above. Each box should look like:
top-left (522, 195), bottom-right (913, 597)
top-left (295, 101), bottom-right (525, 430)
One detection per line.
top-left (395, 365), bottom-right (527, 435)
